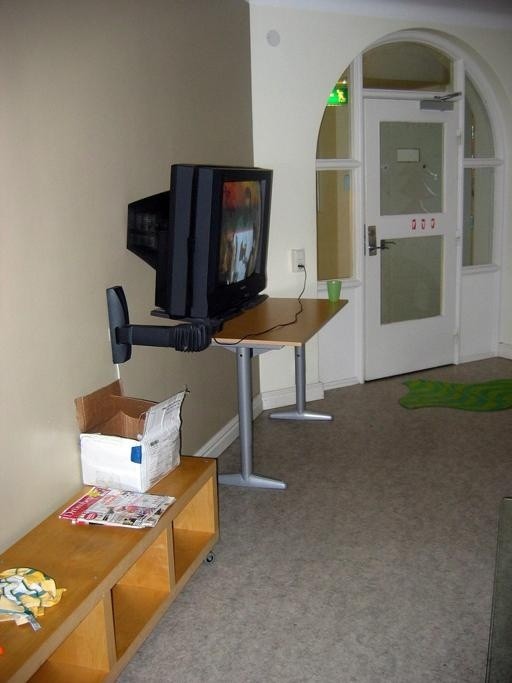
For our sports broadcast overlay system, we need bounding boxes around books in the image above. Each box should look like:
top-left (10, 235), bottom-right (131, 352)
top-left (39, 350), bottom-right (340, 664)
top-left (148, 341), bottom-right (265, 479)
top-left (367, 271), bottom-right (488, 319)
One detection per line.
top-left (71, 495), bottom-right (178, 529)
top-left (57, 486), bottom-right (171, 530)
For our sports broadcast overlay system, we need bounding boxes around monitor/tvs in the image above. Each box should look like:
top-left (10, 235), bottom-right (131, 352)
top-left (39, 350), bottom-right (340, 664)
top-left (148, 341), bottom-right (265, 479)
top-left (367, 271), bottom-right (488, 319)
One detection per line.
top-left (126, 165), bottom-right (274, 318)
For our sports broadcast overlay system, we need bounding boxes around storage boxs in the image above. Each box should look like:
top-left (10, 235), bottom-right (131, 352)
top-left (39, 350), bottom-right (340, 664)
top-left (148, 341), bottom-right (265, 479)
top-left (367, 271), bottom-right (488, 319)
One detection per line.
top-left (75, 380), bottom-right (191, 491)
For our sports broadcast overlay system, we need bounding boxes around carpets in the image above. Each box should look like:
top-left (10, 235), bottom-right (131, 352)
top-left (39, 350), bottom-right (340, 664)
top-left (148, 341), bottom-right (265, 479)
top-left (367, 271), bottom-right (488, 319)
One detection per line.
top-left (398, 376), bottom-right (512, 413)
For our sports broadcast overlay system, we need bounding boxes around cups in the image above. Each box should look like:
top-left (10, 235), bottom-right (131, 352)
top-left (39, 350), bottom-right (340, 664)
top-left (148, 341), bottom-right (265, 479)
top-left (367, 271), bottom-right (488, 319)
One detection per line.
top-left (326, 280), bottom-right (342, 304)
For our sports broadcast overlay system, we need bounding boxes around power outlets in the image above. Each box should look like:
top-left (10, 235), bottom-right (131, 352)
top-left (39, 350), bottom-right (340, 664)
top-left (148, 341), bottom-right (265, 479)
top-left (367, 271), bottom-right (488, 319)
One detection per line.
top-left (292, 248), bottom-right (306, 272)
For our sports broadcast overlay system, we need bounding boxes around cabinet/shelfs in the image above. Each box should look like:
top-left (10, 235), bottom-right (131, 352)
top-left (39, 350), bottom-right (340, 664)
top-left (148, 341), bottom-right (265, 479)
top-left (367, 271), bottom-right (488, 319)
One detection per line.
top-left (1, 453), bottom-right (223, 683)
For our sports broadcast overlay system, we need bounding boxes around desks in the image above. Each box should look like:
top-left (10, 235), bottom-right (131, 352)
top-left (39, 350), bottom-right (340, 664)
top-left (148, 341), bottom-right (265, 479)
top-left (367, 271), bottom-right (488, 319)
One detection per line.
top-left (209, 297), bottom-right (352, 491)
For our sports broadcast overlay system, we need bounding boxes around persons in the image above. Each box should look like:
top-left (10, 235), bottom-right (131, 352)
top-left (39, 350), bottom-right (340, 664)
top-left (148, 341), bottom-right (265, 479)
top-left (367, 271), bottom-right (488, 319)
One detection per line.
top-left (224, 185), bottom-right (256, 284)
